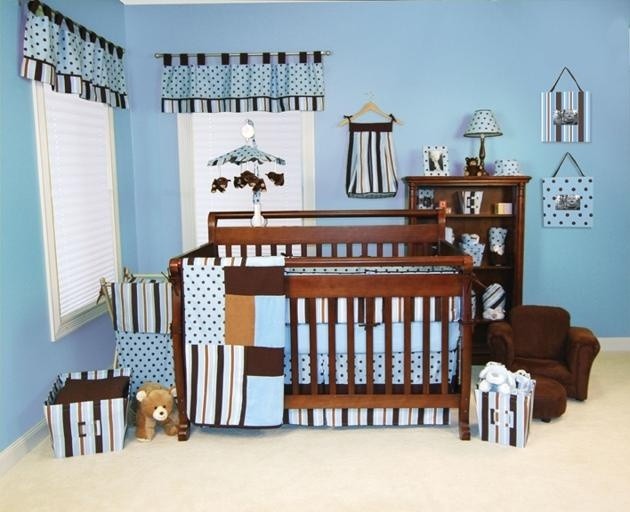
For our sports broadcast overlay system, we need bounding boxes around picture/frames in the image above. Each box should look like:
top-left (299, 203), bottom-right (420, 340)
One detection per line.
top-left (422, 144), bottom-right (449, 176)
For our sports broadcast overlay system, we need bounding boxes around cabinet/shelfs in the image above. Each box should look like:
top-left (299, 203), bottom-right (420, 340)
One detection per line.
top-left (399, 175), bottom-right (533, 367)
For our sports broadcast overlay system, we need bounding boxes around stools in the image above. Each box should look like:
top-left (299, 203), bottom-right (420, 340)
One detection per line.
top-left (510, 376), bottom-right (567, 422)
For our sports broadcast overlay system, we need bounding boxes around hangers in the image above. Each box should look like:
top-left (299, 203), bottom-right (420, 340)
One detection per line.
top-left (337, 99), bottom-right (403, 129)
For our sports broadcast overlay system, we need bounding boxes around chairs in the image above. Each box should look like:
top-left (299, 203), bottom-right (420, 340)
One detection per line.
top-left (483, 304), bottom-right (600, 402)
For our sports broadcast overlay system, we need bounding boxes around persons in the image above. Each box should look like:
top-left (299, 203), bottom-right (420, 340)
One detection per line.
top-left (561, 109), bottom-right (577, 125)
top-left (563, 194), bottom-right (579, 211)
top-left (429, 150), bottom-right (443, 171)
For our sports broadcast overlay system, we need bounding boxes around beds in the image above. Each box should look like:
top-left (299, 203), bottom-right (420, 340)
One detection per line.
top-left (167, 203), bottom-right (477, 443)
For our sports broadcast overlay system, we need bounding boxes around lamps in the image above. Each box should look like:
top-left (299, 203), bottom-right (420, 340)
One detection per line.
top-left (464, 108), bottom-right (503, 173)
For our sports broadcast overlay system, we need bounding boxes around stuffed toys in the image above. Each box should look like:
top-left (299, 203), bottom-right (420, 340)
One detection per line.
top-left (477, 360), bottom-right (533, 396)
top-left (463, 156), bottom-right (482, 177)
top-left (134, 380), bottom-right (178, 441)
top-left (207, 122), bottom-right (287, 226)
